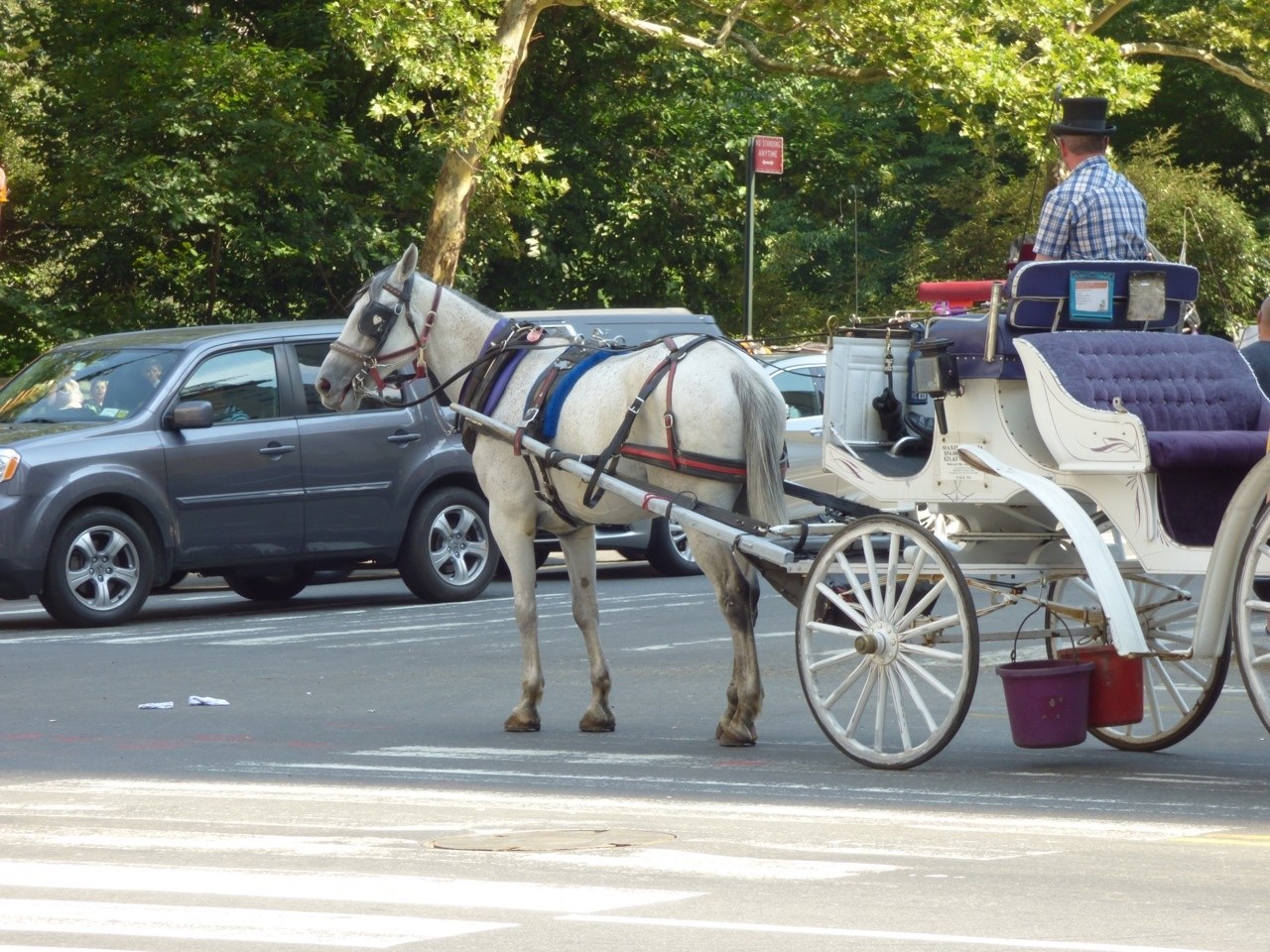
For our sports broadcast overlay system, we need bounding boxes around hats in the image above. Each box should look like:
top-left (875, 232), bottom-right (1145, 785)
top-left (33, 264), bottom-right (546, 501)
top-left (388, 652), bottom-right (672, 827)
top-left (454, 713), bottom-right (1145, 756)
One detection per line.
top-left (1050, 98), bottom-right (1117, 136)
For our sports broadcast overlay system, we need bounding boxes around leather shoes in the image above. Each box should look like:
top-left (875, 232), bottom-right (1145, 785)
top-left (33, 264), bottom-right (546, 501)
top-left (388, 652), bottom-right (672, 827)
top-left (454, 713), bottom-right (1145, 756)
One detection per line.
top-left (904, 411), bottom-right (934, 443)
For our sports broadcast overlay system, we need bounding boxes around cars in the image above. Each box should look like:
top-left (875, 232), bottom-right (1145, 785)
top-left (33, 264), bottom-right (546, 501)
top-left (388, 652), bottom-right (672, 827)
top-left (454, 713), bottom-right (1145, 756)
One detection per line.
top-left (745, 352), bottom-right (916, 521)
top-left (492, 305), bottom-right (727, 575)
top-left (0, 318), bottom-right (497, 627)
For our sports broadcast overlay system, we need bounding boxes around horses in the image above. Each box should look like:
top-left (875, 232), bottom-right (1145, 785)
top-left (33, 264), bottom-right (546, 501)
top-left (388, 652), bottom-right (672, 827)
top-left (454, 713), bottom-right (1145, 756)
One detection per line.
top-left (314, 243), bottom-right (791, 745)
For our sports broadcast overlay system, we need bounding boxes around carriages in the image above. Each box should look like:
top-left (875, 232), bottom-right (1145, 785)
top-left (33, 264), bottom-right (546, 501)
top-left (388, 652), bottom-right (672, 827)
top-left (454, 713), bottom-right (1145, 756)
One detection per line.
top-left (315, 241), bottom-right (1270, 768)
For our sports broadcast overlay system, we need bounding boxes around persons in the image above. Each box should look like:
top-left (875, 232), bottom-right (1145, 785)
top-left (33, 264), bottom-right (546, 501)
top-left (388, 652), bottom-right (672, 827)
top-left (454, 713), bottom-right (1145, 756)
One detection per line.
top-left (43, 358), bottom-right (252, 424)
top-left (904, 96), bottom-right (1151, 441)
top-left (1237, 294), bottom-right (1270, 636)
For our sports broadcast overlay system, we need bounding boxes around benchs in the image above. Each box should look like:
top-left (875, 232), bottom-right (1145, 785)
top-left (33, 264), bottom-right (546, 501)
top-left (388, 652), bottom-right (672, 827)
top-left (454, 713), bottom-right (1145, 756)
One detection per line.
top-left (919, 257), bottom-right (1270, 468)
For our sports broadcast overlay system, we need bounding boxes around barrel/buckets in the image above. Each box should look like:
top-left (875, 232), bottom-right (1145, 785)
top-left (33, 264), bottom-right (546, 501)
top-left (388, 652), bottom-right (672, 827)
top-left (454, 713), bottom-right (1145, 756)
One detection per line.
top-left (1057, 645), bottom-right (1144, 728)
top-left (995, 602), bottom-right (1096, 750)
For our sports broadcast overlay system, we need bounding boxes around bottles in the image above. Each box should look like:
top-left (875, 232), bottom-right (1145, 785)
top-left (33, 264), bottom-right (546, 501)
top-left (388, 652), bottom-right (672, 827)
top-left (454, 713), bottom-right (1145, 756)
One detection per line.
top-left (905, 321), bottom-right (928, 404)
top-left (872, 386), bottom-right (916, 453)
